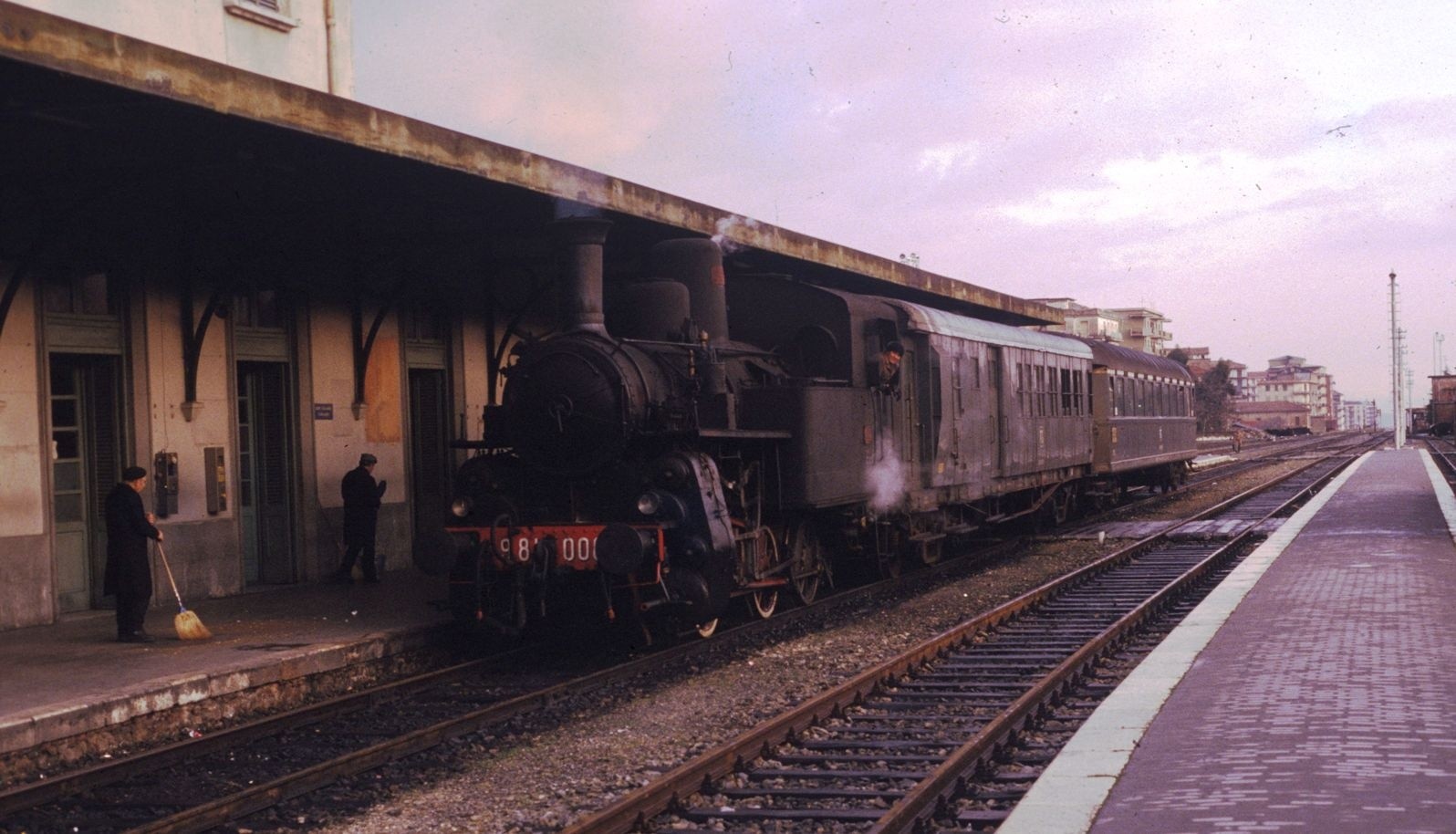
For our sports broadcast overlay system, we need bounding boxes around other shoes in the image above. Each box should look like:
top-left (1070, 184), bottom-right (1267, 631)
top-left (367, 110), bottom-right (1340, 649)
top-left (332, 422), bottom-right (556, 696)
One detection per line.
top-left (338, 573), bottom-right (354, 582)
top-left (364, 577), bottom-right (380, 585)
top-left (117, 629), bottom-right (154, 641)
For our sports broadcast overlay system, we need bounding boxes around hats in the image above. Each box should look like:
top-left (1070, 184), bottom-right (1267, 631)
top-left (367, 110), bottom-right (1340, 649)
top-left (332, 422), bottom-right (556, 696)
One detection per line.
top-left (124, 466), bottom-right (147, 481)
top-left (884, 341), bottom-right (904, 356)
top-left (360, 453), bottom-right (377, 463)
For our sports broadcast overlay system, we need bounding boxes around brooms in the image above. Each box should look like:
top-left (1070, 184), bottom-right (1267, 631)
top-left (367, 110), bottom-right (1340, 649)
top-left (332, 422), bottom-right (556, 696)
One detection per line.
top-left (318, 498), bottom-right (361, 584)
top-left (156, 541), bottom-right (213, 641)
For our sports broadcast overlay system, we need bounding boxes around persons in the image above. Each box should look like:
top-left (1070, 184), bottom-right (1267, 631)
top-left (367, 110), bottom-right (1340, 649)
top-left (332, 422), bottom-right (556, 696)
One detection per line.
top-left (341, 453), bottom-right (385, 586)
top-left (1230, 429), bottom-right (1241, 451)
top-left (103, 466), bottom-right (165, 643)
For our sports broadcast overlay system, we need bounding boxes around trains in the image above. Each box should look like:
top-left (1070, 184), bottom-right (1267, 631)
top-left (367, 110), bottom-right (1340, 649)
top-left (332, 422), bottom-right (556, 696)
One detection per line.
top-left (409, 216), bottom-right (1200, 646)
